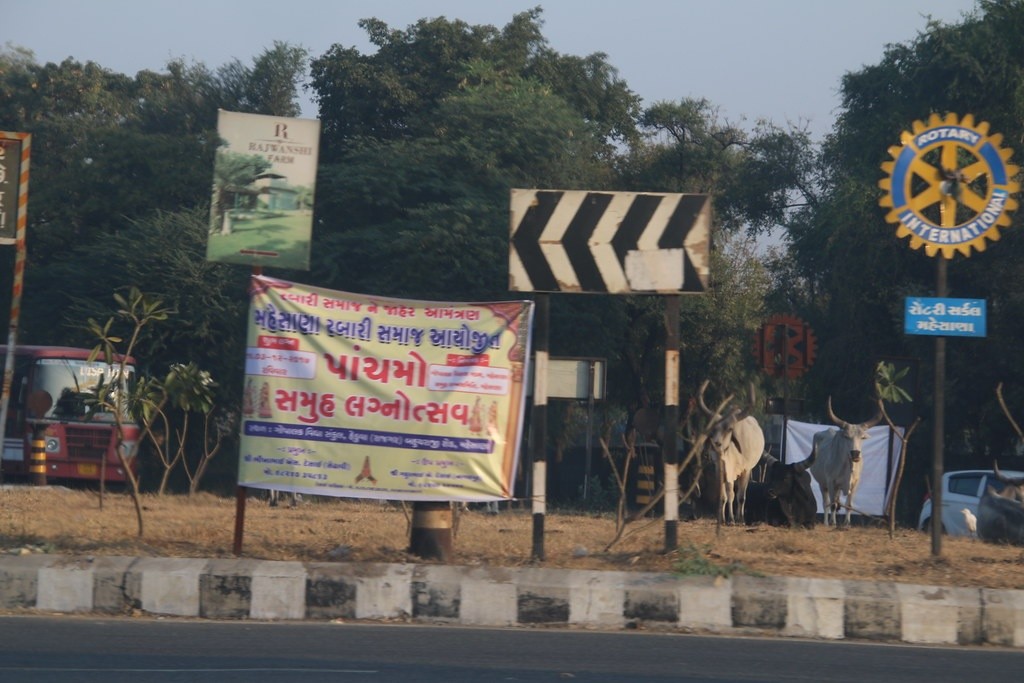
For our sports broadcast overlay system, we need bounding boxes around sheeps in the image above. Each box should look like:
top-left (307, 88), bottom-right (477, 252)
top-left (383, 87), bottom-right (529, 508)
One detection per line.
top-left (744, 441), bottom-right (817, 530)
top-left (696, 378), bottom-right (766, 528)
top-left (809, 393), bottom-right (885, 528)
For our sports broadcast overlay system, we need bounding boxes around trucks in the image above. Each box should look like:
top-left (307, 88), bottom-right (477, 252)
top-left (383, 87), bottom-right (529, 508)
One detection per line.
top-left (0, 344), bottom-right (139, 492)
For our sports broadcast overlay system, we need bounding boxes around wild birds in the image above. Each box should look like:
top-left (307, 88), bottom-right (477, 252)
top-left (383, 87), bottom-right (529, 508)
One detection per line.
top-left (959, 507), bottom-right (977, 537)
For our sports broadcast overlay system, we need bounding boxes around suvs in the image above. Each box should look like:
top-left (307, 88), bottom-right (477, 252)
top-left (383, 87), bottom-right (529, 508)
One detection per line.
top-left (918, 469), bottom-right (1024, 542)
